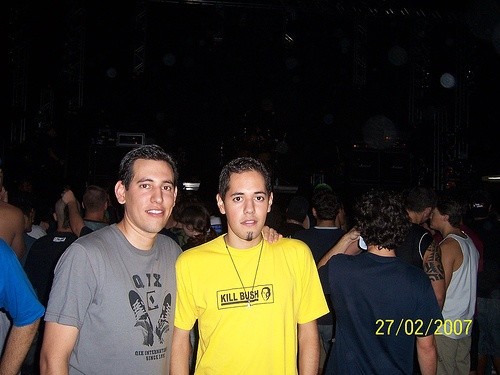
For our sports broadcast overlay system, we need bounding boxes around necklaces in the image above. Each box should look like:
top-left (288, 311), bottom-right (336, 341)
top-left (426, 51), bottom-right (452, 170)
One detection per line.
top-left (224, 234), bottom-right (264, 307)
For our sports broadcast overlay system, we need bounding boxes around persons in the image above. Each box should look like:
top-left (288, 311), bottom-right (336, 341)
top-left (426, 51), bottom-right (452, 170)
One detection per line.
top-left (26, 197), bottom-right (81, 341)
top-left (40, 146), bottom-right (282, 375)
top-left (168, 156), bottom-right (330, 375)
top-left (0, 239), bottom-right (45, 375)
top-left (0, 150), bottom-right (500, 375)
top-left (316, 190), bottom-right (444, 375)
top-left (393, 187), bottom-right (446, 314)
top-left (429, 198), bottom-right (480, 375)
